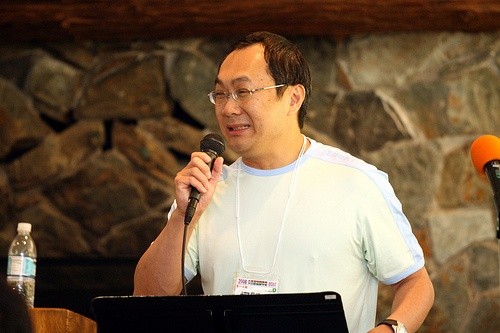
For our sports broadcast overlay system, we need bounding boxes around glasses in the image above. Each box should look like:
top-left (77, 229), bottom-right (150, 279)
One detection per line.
top-left (208, 83), bottom-right (286, 105)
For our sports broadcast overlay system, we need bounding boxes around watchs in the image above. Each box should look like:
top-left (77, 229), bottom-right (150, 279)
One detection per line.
top-left (377, 319), bottom-right (408, 333)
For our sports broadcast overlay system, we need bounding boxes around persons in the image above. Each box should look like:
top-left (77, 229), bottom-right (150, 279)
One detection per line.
top-left (133, 31), bottom-right (434, 333)
top-left (0, 276), bottom-right (37, 333)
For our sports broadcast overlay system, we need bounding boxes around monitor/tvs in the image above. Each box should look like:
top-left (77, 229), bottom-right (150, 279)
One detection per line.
top-left (92, 292), bottom-right (349, 333)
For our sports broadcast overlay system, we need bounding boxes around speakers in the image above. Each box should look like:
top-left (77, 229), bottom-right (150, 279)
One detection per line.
top-left (0, 257), bottom-right (136, 322)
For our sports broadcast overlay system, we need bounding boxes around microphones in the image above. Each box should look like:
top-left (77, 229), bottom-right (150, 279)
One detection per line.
top-left (470, 135), bottom-right (500, 218)
top-left (184, 133), bottom-right (225, 225)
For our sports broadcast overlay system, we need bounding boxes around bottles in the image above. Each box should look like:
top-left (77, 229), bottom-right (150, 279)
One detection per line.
top-left (7, 222), bottom-right (37, 308)
